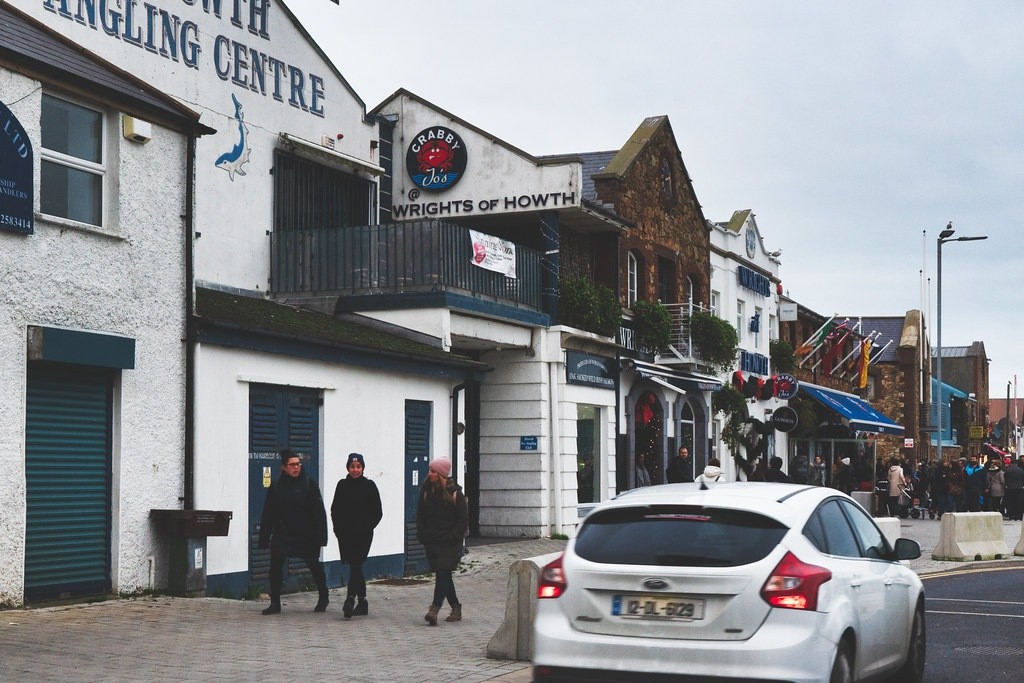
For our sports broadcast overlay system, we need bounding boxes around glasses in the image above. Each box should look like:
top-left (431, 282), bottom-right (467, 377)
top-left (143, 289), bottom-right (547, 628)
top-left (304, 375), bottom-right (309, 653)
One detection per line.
top-left (285, 462), bottom-right (302, 468)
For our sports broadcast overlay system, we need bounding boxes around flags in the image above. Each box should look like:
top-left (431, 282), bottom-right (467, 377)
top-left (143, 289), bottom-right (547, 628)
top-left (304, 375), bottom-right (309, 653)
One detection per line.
top-left (821, 329), bottom-right (852, 377)
top-left (822, 321), bottom-right (847, 345)
top-left (795, 320), bottom-right (833, 358)
top-left (858, 335), bottom-right (871, 389)
top-left (854, 338), bottom-right (876, 365)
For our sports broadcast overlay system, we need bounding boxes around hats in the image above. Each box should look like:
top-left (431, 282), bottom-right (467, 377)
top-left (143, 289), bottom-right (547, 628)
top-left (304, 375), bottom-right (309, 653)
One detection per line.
top-left (346, 453), bottom-right (365, 470)
top-left (428, 457), bottom-right (451, 477)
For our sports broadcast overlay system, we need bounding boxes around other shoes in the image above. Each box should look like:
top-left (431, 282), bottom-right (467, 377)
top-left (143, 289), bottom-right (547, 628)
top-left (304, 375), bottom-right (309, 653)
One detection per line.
top-left (260, 603), bottom-right (281, 615)
top-left (313, 599), bottom-right (331, 612)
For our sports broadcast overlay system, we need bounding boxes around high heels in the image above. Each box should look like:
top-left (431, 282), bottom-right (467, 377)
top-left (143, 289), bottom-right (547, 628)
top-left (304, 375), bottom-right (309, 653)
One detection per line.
top-left (350, 600), bottom-right (368, 615)
top-left (343, 596), bottom-right (354, 618)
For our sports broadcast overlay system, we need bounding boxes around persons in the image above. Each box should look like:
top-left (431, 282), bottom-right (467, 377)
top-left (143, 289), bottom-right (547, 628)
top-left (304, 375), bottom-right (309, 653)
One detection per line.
top-left (416, 456), bottom-right (469, 626)
top-left (694, 459), bottom-right (725, 485)
top-left (768, 455), bottom-right (791, 481)
top-left (834, 447), bottom-right (1024, 521)
top-left (258, 450), bottom-right (331, 616)
top-left (665, 446), bottom-right (694, 484)
top-left (808, 454), bottom-right (825, 486)
top-left (791, 455), bottom-right (809, 484)
top-left (331, 452), bottom-right (383, 619)
top-left (636, 453), bottom-right (651, 488)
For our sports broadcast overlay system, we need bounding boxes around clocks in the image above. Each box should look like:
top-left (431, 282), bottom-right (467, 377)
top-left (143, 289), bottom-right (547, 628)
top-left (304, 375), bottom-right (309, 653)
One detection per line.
top-left (746, 222), bottom-right (757, 259)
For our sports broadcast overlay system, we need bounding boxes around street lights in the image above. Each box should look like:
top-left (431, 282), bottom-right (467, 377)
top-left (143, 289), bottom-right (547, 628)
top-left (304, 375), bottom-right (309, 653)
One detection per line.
top-left (936, 220), bottom-right (989, 460)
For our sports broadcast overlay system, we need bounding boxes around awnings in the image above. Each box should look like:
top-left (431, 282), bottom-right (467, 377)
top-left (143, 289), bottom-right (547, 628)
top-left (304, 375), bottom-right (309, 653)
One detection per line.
top-left (797, 380), bottom-right (906, 431)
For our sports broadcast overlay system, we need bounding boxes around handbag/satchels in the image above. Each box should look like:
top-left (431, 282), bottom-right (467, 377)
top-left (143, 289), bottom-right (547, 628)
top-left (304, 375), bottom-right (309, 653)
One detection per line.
top-left (949, 485), bottom-right (961, 495)
top-left (984, 489), bottom-right (990, 496)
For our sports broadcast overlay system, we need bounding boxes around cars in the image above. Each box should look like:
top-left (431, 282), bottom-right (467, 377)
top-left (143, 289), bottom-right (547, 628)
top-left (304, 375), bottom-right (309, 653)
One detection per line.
top-left (531, 480), bottom-right (927, 682)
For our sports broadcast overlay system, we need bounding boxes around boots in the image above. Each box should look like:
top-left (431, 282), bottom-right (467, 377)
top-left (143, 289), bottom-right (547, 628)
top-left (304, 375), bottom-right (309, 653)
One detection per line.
top-left (445, 603), bottom-right (462, 621)
top-left (425, 602), bottom-right (441, 625)
